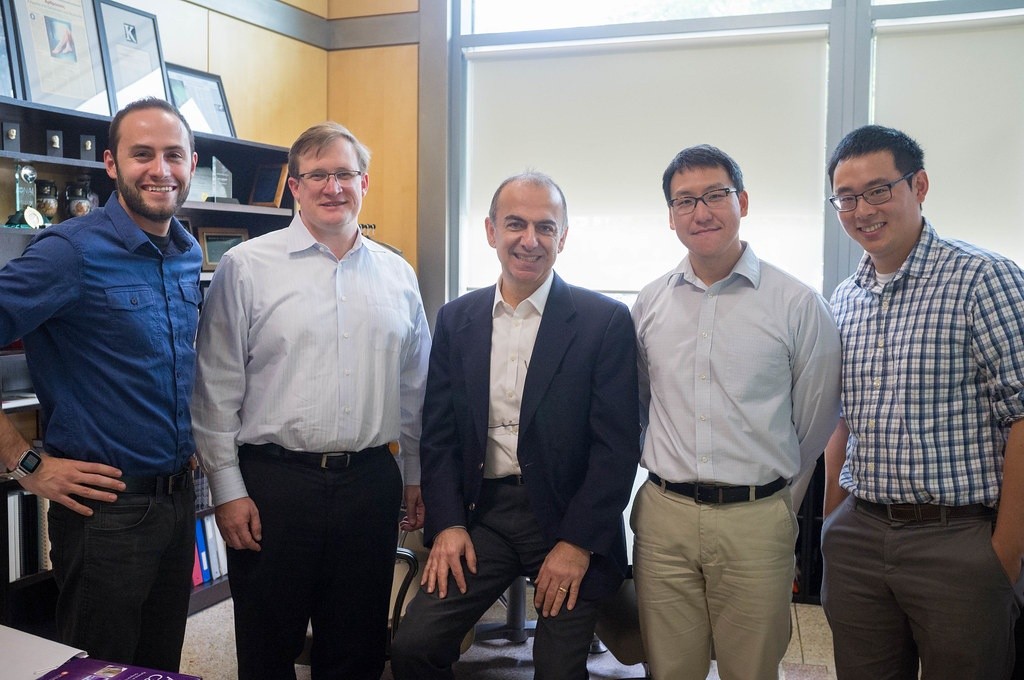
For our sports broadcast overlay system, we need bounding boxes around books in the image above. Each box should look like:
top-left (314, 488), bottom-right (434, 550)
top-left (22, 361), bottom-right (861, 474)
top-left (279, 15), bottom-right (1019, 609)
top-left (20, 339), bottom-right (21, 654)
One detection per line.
top-left (4, 488), bottom-right (53, 581)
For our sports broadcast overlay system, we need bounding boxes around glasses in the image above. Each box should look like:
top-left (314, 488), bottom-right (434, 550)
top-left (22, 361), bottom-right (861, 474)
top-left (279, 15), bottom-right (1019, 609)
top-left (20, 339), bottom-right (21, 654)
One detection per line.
top-left (668, 187), bottom-right (737, 214)
top-left (299, 170), bottom-right (362, 188)
top-left (828, 172), bottom-right (913, 212)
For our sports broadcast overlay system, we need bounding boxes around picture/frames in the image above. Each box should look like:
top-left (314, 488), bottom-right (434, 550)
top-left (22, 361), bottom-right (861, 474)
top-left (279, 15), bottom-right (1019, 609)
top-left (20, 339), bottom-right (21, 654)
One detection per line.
top-left (247, 162), bottom-right (288, 208)
top-left (197, 226), bottom-right (249, 272)
top-left (164, 62), bottom-right (238, 139)
top-left (92, 0), bottom-right (173, 117)
top-left (0, 0), bottom-right (24, 100)
top-left (11, 0), bottom-right (111, 116)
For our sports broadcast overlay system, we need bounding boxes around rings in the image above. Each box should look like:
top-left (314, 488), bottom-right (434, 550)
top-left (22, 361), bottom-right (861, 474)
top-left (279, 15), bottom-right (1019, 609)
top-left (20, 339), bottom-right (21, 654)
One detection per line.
top-left (558, 586), bottom-right (568, 593)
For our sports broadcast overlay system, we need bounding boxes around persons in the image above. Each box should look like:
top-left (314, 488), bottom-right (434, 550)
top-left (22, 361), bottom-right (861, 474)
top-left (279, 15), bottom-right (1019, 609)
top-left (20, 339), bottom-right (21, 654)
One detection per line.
top-left (820, 126), bottom-right (1024, 680)
top-left (389, 172), bottom-right (641, 680)
top-left (630, 145), bottom-right (842, 680)
top-left (0, 97), bottom-right (205, 674)
top-left (191, 124), bottom-right (433, 680)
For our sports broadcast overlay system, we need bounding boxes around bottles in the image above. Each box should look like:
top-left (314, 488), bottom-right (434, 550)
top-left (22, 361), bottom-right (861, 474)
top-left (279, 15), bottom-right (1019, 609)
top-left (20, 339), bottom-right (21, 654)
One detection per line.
top-left (35, 172), bottom-right (100, 222)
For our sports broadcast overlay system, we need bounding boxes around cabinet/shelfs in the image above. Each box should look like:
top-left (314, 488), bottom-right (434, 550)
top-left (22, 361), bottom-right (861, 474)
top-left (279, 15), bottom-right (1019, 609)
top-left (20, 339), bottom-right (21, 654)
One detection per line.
top-left (177, 131), bottom-right (299, 618)
top-left (0, 95), bottom-right (116, 638)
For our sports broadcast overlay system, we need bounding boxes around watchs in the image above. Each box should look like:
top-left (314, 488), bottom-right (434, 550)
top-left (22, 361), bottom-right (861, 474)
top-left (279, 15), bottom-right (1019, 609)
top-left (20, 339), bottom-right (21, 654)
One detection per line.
top-left (0, 444), bottom-right (42, 482)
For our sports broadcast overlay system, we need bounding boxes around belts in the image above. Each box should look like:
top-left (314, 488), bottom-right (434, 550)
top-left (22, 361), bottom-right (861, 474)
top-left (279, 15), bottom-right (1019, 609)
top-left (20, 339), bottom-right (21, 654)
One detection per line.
top-left (493, 475), bottom-right (526, 486)
top-left (78, 463), bottom-right (194, 496)
top-left (648, 472), bottom-right (787, 505)
top-left (260, 443), bottom-right (389, 469)
top-left (850, 496), bottom-right (998, 528)
